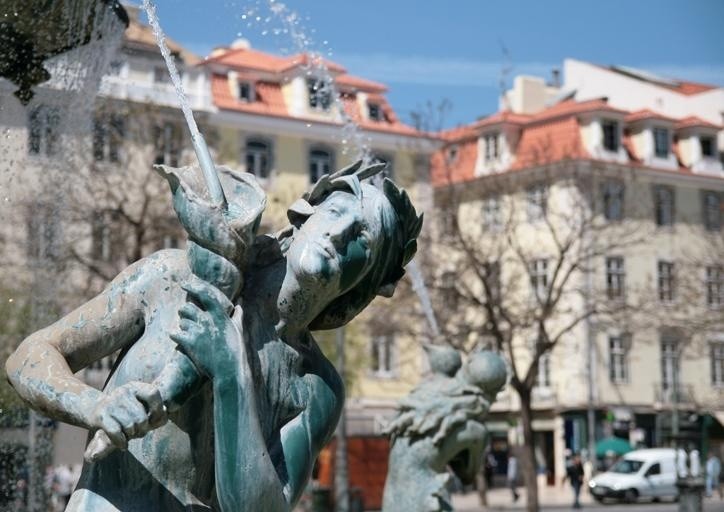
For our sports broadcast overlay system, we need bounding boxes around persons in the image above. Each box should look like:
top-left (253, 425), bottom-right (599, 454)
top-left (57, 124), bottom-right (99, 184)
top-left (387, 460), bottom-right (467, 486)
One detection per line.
top-left (678, 444), bottom-right (689, 477)
top-left (705, 449), bottom-right (720, 497)
top-left (599, 449), bottom-right (616, 470)
top-left (689, 443), bottom-right (700, 477)
top-left (6, 161), bottom-right (422, 512)
top-left (507, 447), bottom-right (520, 502)
top-left (484, 444), bottom-right (494, 487)
top-left (567, 453), bottom-right (584, 507)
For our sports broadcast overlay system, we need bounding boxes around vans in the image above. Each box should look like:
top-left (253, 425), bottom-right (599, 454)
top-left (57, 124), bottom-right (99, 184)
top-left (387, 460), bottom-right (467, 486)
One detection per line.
top-left (587, 447), bottom-right (706, 504)
